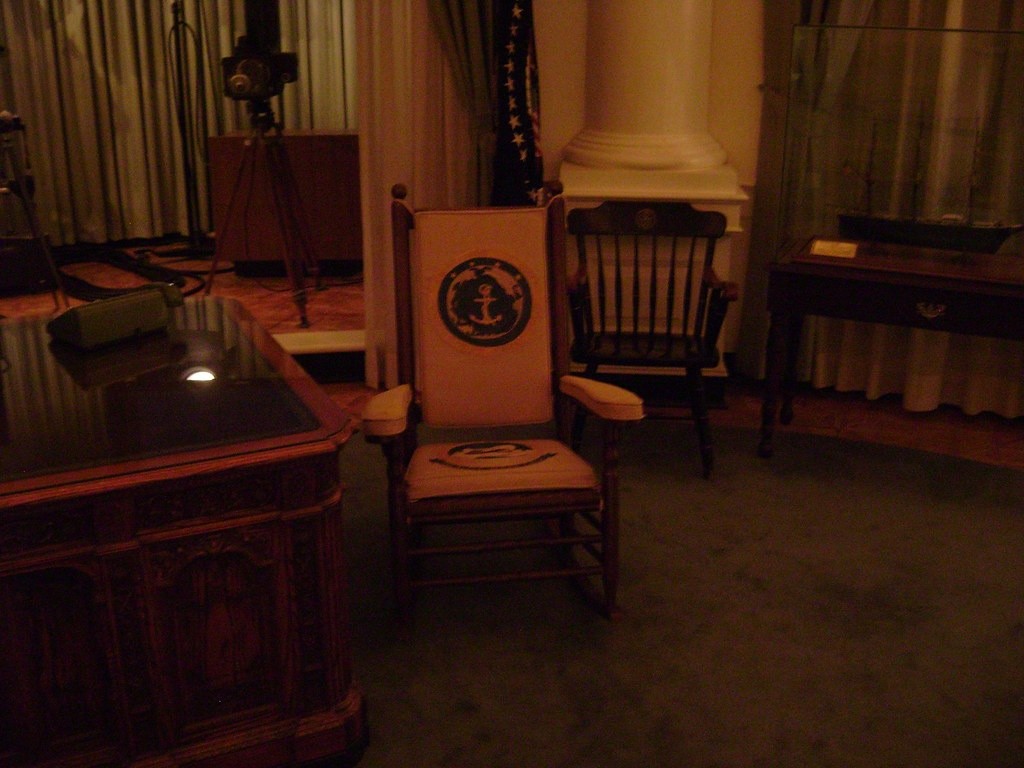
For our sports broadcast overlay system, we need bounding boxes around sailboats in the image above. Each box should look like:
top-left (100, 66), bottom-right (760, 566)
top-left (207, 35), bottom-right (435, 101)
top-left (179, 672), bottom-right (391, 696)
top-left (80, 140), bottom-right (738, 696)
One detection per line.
top-left (833, 88), bottom-right (1023, 257)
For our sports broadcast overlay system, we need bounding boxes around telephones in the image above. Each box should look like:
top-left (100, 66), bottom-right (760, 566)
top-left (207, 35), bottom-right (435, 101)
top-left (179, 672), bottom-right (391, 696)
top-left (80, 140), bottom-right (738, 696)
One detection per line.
top-left (46, 282), bottom-right (183, 349)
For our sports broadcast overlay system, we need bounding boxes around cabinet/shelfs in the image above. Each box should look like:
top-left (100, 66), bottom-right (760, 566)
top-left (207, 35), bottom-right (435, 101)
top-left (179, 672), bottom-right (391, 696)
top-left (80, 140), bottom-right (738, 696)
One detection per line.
top-left (209, 127), bottom-right (361, 276)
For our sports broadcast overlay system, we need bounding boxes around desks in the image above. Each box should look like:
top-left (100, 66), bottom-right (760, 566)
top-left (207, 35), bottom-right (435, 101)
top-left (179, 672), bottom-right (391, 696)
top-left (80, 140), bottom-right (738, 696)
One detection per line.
top-left (0, 294), bottom-right (371, 768)
top-left (752, 235), bottom-right (1024, 458)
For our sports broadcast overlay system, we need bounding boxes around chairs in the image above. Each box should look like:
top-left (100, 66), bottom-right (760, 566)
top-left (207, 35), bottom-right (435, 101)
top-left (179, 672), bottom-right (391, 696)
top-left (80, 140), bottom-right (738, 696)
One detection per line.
top-left (564, 199), bottom-right (739, 481)
top-left (349, 179), bottom-right (647, 641)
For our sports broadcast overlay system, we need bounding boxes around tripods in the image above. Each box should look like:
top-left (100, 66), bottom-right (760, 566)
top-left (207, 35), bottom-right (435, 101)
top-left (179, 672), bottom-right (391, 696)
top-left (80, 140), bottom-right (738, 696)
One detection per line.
top-left (203, 101), bottom-right (324, 328)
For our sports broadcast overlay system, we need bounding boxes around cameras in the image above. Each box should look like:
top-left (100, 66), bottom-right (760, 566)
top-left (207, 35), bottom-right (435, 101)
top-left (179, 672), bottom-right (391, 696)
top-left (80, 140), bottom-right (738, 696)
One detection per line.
top-left (220, 32), bottom-right (300, 102)
